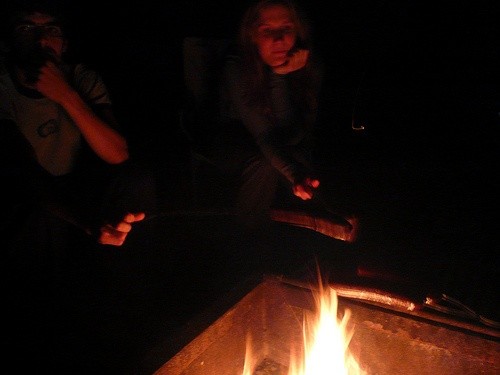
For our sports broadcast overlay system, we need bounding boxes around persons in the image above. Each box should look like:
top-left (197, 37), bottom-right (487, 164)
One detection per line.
top-left (0, 0), bottom-right (147, 273)
top-left (213, 0), bottom-right (339, 209)
